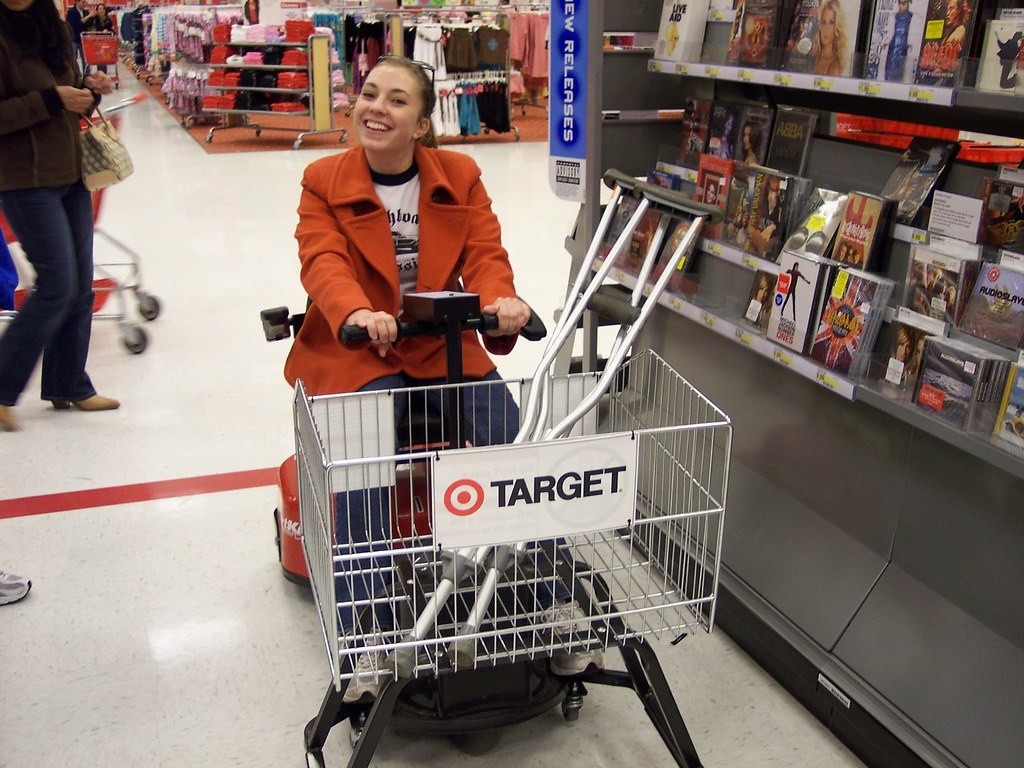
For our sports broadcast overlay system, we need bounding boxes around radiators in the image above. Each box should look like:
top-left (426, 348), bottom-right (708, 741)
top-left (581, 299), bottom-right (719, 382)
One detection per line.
top-left (362, 12), bottom-right (378, 24)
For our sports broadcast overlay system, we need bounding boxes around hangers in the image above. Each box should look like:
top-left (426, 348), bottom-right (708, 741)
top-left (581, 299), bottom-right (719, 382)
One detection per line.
top-left (108, 5), bottom-right (138, 13)
top-left (150, 6), bottom-right (242, 16)
top-left (434, 71), bottom-right (506, 83)
top-left (402, 17), bottom-right (502, 28)
top-left (311, 6), bottom-right (338, 14)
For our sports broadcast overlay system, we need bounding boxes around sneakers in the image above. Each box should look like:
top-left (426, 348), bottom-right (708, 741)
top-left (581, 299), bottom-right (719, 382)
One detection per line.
top-left (0, 570), bottom-right (32, 606)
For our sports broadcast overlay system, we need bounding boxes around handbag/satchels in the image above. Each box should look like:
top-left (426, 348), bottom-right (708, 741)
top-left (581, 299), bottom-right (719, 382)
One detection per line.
top-left (78, 106), bottom-right (135, 192)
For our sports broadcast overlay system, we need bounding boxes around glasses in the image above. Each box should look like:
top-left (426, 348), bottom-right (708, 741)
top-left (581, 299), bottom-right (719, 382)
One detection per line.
top-left (377, 56), bottom-right (436, 93)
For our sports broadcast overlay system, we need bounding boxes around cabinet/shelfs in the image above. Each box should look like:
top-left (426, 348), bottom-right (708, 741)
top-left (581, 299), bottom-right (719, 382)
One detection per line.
top-left (553, 0), bottom-right (1024, 768)
top-left (204, 34), bottom-right (347, 150)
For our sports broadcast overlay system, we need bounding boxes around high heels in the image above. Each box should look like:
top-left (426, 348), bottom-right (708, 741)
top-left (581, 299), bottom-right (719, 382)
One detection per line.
top-left (51, 393), bottom-right (121, 411)
top-left (0, 404), bottom-right (22, 429)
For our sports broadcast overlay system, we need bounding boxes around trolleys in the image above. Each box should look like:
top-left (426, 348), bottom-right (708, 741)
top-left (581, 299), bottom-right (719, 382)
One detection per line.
top-left (262, 291), bottom-right (731, 767)
top-left (80, 30), bottom-right (121, 92)
top-left (0, 91), bottom-right (159, 355)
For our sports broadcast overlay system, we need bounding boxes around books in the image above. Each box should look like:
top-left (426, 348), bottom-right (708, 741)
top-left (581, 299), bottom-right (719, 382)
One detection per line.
top-left (603, 0), bottom-right (1024, 448)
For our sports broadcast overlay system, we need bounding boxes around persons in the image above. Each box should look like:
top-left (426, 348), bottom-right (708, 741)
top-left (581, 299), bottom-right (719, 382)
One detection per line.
top-left (742, 121), bottom-right (763, 164)
top-left (67, 0), bottom-right (115, 78)
top-left (994, 27), bottom-right (1023, 88)
top-left (284, 57), bottom-right (606, 706)
top-left (834, 241), bottom-right (864, 267)
top-left (881, 324), bottom-right (916, 391)
top-left (812, 0), bottom-right (848, 76)
top-left (0, 0), bottom-right (124, 434)
top-left (907, 262), bottom-right (957, 320)
top-left (885, 0), bottom-right (913, 81)
top-left (781, 261), bottom-right (811, 320)
top-left (706, 183), bottom-right (717, 203)
top-left (0, 569), bottom-right (32, 607)
top-left (940, 0), bottom-right (971, 54)
